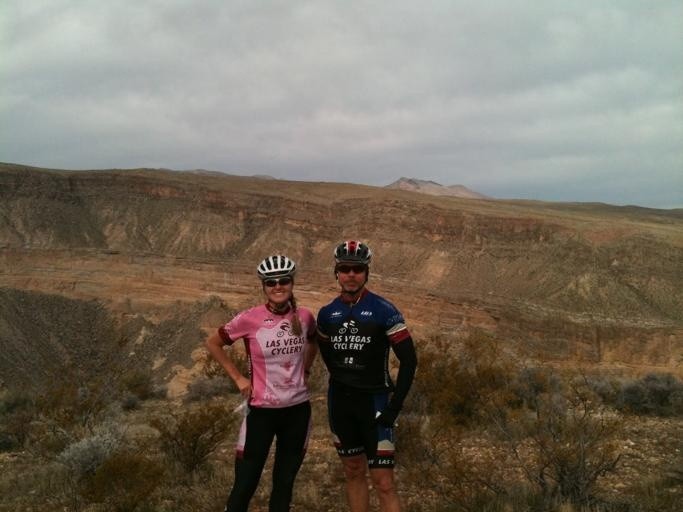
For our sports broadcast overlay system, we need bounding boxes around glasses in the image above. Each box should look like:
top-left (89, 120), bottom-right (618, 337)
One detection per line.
top-left (337, 264), bottom-right (366, 273)
top-left (264, 279), bottom-right (292, 287)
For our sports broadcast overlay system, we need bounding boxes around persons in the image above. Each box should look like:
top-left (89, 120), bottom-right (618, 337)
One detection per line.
top-left (310, 241), bottom-right (417, 511)
top-left (204, 254), bottom-right (319, 512)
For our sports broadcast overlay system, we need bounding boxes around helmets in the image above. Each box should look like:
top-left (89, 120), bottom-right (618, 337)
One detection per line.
top-left (335, 239), bottom-right (372, 264)
top-left (257, 255), bottom-right (297, 278)
